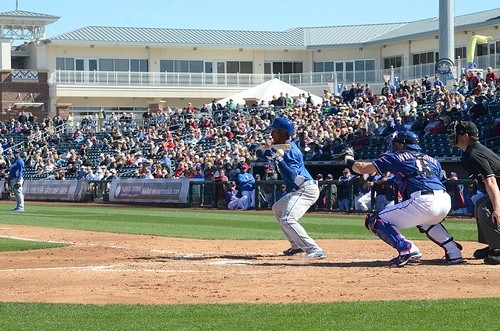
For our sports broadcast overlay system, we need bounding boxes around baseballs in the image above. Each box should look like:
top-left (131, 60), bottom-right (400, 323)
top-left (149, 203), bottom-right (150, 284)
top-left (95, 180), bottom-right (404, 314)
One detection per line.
top-left (276, 148), bottom-right (285, 157)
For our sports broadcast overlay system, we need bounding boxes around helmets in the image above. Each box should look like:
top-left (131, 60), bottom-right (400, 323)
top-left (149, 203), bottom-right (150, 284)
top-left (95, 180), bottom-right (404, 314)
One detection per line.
top-left (268, 118), bottom-right (294, 136)
top-left (396, 131), bottom-right (422, 150)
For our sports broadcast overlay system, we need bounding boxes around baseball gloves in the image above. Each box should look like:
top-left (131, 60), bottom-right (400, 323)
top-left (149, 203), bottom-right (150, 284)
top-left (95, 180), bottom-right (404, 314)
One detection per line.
top-left (366, 180), bottom-right (404, 201)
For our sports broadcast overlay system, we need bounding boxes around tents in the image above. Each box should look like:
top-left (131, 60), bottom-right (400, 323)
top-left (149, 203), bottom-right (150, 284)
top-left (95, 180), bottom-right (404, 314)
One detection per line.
top-left (203, 78), bottom-right (323, 107)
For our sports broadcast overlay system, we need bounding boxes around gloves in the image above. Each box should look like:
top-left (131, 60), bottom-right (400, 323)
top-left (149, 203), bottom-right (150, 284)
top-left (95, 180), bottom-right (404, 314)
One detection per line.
top-left (15, 181), bottom-right (21, 187)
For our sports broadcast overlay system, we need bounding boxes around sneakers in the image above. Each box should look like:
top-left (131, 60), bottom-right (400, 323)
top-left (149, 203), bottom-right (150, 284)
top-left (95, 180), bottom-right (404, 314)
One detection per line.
top-left (390, 246), bottom-right (421, 267)
top-left (445, 257), bottom-right (464, 265)
top-left (305, 251), bottom-right (327, 260)
top-left (282, 248), bottom-right (304, 256)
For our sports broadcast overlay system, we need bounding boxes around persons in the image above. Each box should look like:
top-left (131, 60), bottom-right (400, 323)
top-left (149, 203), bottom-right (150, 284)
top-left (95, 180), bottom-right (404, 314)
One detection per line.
top-left (441, 170), bottom-right (488, 214)
top-left (8, 148), bottom-right (24, 211)
top-left (452, 121), bottom-right (500, 264)
top-left (340, 131), bottom-right (464, 269)
top-left (135, 99), bottom-right (250, 181)
top-left (242, 92), bottom-right (410, 158)
top-left (223, 162), bottom-right (255, 209)
top-left (342, 66), bottom-right (500, 147)
top-left (315, 167), bottom-right (402, 213)
top-left (0, 111), bottom-right (136, 193)
top-left (267, 117), bottom-right (326, 259)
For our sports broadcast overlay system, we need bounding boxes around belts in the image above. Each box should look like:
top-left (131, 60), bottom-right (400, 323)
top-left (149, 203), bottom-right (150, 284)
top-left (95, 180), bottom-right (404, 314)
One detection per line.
top-left (421, 189), bottom-right (446, 195)
top-left (9, 177), bottom-right (17, 181)
top-left (292, 180), bottom-right (317, 191)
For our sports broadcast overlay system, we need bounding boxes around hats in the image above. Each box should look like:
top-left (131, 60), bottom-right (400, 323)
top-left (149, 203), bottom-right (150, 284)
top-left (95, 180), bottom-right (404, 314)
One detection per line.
top-left (14, 148), bottom-right (20, 153)
top-left (456, 121), bottom-right (479, 141)
top-left (230, 181), bottom-right (236, 186)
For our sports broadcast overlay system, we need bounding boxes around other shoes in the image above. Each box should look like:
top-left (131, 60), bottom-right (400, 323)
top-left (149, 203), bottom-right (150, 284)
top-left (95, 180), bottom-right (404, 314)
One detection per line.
top-left (483, 250), bottom-right (500, 265)
top-left (13, 208), bottom-right (24, 212)
top-left (473, 246), bottom-right (493, 259)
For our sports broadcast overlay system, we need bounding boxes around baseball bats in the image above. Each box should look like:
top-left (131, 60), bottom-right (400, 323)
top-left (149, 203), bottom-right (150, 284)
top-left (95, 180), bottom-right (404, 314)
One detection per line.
top-left (253, 143), bottom-right (291, 151)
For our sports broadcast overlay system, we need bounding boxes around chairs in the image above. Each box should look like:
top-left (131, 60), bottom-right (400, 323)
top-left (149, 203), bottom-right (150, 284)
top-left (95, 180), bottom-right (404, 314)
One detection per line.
top-left (0, 120), bottom-right (150, 184)
